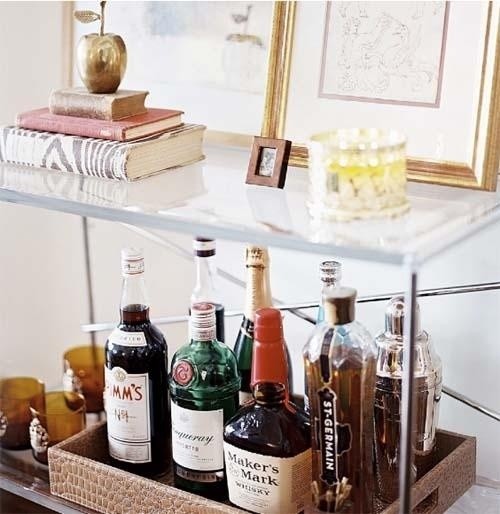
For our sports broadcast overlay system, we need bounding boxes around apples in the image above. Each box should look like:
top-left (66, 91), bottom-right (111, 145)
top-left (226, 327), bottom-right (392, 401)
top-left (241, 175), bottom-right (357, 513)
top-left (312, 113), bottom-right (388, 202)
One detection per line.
top-left (72, 1), bottom-right (128, 94)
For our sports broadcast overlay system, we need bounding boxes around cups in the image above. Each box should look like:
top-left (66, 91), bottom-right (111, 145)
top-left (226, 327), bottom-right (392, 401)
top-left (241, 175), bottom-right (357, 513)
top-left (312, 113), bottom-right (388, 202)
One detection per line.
top-left (306, 126), bottom-right (411, 223)
top-left (28, 391), bottom-right (87, 466)
top-left (63, 346), bottom-right (106, 413)
top-left (0, 376), bottom-right (45, 451)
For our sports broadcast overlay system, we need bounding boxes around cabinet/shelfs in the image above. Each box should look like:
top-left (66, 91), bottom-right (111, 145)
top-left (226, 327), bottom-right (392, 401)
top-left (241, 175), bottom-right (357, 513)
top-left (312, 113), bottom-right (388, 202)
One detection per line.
top-left (1, 146), bottom-right (500, 513)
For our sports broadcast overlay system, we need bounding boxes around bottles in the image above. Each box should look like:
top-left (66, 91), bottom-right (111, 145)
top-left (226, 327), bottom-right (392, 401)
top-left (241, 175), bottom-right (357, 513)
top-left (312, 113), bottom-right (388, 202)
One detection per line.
top-left (304, 260), bottom-right (343, 418)
top-left (308, 286), bottom-right (377, 514)
top-left (223, 380), bottom-right (313, 514)
top-left (104, 248), bottom-right (170, 480)
top-left (374, 294), bottom-right (443, 506)
top-left (168, 303), bottom-right (241, 502)
top-left (242, 307), bottom-right (311, 423)
top-left (188, 237), bottom-right (224, 346)
top-left (232, 244), bottom-right (293, 409)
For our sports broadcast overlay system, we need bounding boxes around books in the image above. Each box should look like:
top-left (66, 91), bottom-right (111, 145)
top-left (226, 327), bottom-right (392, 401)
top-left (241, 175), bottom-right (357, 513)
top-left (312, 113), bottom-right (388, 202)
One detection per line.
top-left (48, 85), bottom-right (150, 123)
top-left (0, 123), bottom-right (206, 183)
top-left (15, 106), bottom-right (186, 142)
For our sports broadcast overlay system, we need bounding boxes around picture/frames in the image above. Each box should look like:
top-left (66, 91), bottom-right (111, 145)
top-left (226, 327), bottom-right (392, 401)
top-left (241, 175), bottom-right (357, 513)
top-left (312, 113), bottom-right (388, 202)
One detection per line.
top-left (245, 136), bottom-right (291, 189)
top-left (60, 1), bottom-right (285, 151)
top-left (260, 1), bottom-right (498, 193)
